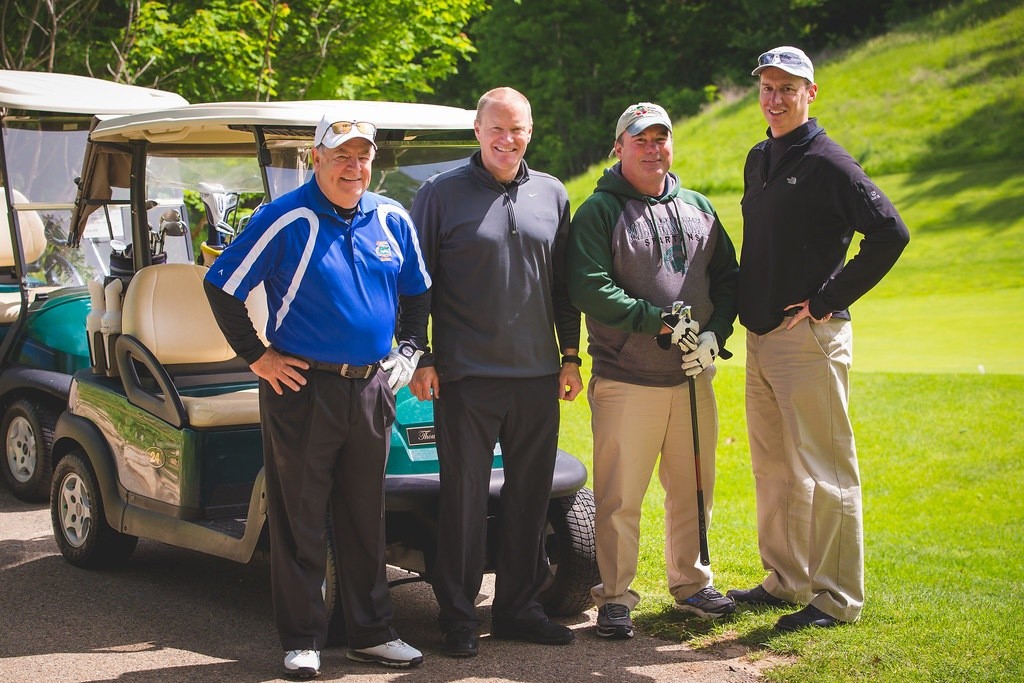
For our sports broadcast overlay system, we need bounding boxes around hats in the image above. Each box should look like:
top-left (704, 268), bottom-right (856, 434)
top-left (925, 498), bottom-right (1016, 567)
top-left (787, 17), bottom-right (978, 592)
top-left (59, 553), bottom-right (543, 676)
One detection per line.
top-left (616, 102), bottom-right (672, 140)
top-left (315, 106), bottom-right (378, 150)
top-left (752, 46), bottom-right (814, 84)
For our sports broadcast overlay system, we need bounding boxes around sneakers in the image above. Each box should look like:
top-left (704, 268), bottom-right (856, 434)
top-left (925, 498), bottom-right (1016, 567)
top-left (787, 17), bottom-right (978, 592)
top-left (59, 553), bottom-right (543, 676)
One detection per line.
top-left (726, 584), bottom-right (797, 607)
top-left (490, 616), bottom-right (574, 645)
top-left (774, 604), bottom-right (845, 631)
top-left (595, 604), bottom-right (635, 639)
top-left (282, 650), bottom-right (321, 677)
top-left (439, 625), bottom-right (479, 657)
top-left (346, 639), bottom-right (423, 667)
top-left (673, 587), bottom-right (735, 620)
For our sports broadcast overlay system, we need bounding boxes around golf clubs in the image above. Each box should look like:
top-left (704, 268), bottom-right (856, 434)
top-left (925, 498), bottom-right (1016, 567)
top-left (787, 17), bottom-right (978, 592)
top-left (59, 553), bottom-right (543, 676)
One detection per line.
top-left (216, 190), bottom-right (269, 243)
top-left (110, 200), bottom-right (189, 255)
top-left (670, 300), bottom-right (713, 567)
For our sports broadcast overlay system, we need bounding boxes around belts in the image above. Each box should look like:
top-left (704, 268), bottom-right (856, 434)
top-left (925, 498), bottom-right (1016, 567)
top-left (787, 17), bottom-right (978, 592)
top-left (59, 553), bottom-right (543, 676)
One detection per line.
top-left (282, 352), bottom-right (388, 379)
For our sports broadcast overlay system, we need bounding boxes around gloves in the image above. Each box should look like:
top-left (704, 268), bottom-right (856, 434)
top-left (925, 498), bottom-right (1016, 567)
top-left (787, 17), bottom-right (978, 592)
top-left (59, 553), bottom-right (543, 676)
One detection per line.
top-left (653, 305), bottom-right (701, 352)
top-left (681, 331), bottom-right (719, 379)
top-left (380, 340), bottom-right (425, 395)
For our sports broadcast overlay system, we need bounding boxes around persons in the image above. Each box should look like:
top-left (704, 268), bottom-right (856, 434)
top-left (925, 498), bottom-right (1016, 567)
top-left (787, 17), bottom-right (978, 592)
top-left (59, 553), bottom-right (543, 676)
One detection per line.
top-left (203, 109), bottom-right (434, 680)
top-left (726, 46), bottom-right (911, 633)
top-left (564, 101), bottom-right (740, 640)
top-left (393, 86), bottom-right (584, 658)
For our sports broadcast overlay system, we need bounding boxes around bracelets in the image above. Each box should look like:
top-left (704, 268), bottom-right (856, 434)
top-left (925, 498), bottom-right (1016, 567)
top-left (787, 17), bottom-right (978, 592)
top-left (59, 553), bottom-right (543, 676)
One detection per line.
top-left (562, 356), bottom-right (581, 367)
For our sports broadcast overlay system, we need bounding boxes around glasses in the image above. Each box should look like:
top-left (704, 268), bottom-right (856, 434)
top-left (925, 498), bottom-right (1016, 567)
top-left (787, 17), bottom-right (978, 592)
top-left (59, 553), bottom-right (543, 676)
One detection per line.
top-left (317, 121), bottom-right (377, 148)
top-left (757, 52), bottom-right (815, 73)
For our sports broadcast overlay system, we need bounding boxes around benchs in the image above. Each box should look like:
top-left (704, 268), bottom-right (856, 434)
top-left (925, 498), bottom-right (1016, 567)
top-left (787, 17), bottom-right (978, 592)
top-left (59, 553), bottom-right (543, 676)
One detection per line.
top-left (0, 187), bottom-right (84, 323)
top-left (115, 263), bottom-right (269, 429)
